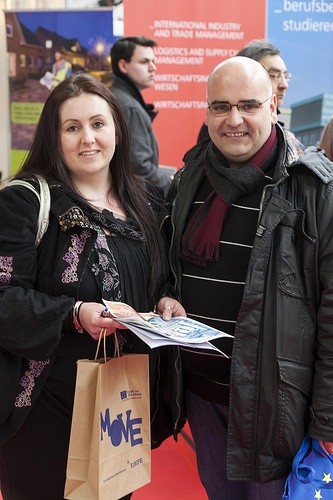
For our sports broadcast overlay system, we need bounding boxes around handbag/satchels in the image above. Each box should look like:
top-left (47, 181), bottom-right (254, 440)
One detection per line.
top-left (282, 436), bottom-right (333, 500)
top-left (64, 326), bottom-right (152, 500)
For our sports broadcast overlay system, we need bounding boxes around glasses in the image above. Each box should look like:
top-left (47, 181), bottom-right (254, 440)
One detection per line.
top-left (207, 96), bottom-right (273, 116)
top-left (269, 69), bottom-right (292, 85)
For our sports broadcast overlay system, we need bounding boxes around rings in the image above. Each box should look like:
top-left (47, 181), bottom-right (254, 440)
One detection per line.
top-left (163, 306), bottom-right (173, 310)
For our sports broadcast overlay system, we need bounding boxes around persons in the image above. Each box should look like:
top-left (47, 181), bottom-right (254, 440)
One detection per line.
top-left (0, 73), bottom-right (187, 500)
top-left (234, 38), bottom-right (333, 162)
top-left (103, 35), bottom-right (174, 201)
top-left (163, 56), bottom-right (333, 500)
top-left (49, 50), bottom-right (73, 84)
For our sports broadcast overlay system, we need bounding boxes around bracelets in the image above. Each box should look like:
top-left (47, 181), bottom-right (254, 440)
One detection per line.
top-left (73, 301), bottom-right (84, 333)
